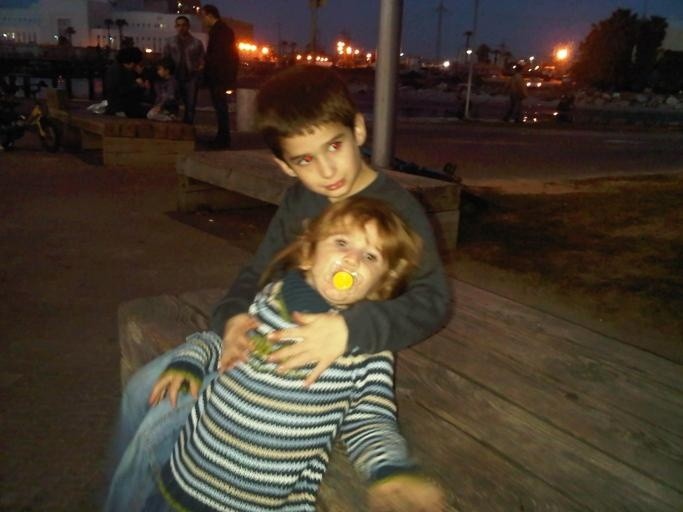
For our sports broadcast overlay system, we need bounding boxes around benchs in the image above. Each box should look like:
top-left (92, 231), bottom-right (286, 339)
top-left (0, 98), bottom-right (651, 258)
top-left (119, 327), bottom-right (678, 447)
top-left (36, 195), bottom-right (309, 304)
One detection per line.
top-left (118, 277), bottom-right (682, 512)
top-left (177, 149), bottom-right (460, 266)
top-left (40, 87), bottom-right (195, 172)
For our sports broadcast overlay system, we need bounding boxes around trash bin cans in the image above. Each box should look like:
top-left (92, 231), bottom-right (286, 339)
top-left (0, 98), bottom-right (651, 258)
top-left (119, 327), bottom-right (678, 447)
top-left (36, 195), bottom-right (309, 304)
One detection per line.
top-left (235, 87), bottom-right (260, 132)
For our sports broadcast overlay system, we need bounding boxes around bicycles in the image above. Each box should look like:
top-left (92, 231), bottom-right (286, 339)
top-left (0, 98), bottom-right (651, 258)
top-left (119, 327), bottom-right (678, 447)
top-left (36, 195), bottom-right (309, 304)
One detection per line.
top-left (1, 79), bottom-right (64, 153)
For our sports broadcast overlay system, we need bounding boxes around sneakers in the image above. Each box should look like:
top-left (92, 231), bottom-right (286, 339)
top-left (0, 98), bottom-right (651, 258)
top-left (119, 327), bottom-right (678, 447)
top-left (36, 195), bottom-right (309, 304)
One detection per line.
top-left (214, 135), bottom-right (232, 148)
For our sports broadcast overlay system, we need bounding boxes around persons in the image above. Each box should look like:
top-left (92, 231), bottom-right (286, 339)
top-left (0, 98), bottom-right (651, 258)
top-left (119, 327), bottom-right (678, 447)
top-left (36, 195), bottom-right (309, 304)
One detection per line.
top-left (98, 47), bottom-right (152, 116)
top-left (501, 63), bottom-right (527, 122)
top-left (159, 16), bottom-right (205, 124)
top-left (98, 62), bottom-right (455, 512)
top-left (144, 60), bottom-right (181, 124)
top-left (139, 195), bottom-right (455, 512)
top-left (44, 35), bottom-right (78, 98)
top-left (182, 4), bottom-right (239, 148)
